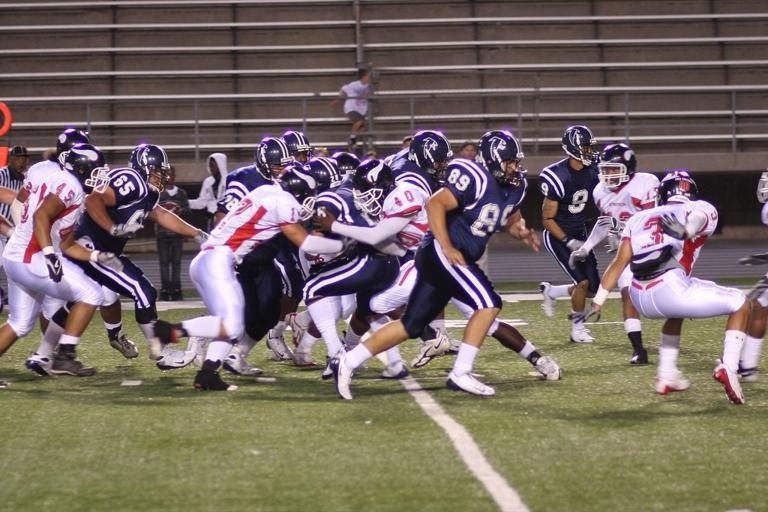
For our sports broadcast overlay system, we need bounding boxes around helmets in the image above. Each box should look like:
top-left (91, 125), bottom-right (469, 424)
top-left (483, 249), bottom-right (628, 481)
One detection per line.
top-left (562, 125), bottom-right (596, 167)
top-left (756, 169), bottom-right (768, 204)
top-left (476, 130), bottom-right (527, 189)
top-left (128, 144), bottom-right (172, 193)
top-left (407, 129), bottom-right (453, 182)
top-left (597, 143), bottom-right (635, 191)
top-left (56, 126), bottom-right (111, 193)
top-left (255, 131), bottom-right (395, 222)
top-left (655, 171), bottom-right (697, 204)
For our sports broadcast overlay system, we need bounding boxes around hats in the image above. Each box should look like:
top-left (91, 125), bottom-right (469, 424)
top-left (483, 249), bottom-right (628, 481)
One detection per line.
top-left (9, 146), bottom-right (28, 156)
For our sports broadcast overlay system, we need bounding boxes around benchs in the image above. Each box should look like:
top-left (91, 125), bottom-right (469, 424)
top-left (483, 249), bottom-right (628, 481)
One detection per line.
top-left (0, 0), bottom-right (768, 164)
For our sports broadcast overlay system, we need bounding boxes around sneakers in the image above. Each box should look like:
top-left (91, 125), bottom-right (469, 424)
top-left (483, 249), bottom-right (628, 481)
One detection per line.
top-left (266, 314), bottom-right (408, 398)
top-left (147, 320), bottom-right (263, 391)
top-left (539, 282), bottom-right (554, 316)
top-left (26, 352), bottom-right (95, 376)
top-left (535, 356), bottom-right (560, 380)
top-left (654, 376), bottom-right (689, 393)
top-left (570, 327), bottom-right (594, 342)
top-left (109, 330), bottom-right (138, 358)
top-left (713, 358), bottom-right (758, 404)
top-left (411, 328), bottom-right (462, 367)
top-left (631, 348), bottom-right (646, 364)
top-left (446, 372), bottom-right (494, 395)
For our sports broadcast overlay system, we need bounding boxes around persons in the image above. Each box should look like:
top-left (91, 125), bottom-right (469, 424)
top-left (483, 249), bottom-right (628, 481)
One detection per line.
top-left (330, 68), bottom-right (370, 148)
top-left (0, 130), bottom-right (768, 404)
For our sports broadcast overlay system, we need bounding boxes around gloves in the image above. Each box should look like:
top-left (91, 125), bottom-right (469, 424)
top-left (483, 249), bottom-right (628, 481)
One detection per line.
top-left (658, 213), bottom-right (687, 240)
top-left (604, 235), bottom-right (619, 253)
top-left (98, 251), bottom-right (123, 272)
top-left (566, 238), bottom-right (587, 271)
top-left (748, 276), bottom-right (768, 299)
top-left (114, 210), bottom-right (144, 236)
top-left (45, 253), bottom-right (63, 282)
top-left (738, 252), bottom-right (768, 264)
top-left (570, 303), bottom-right (600, 323)
top-left (597, 216), bottom-right (626, 231)
